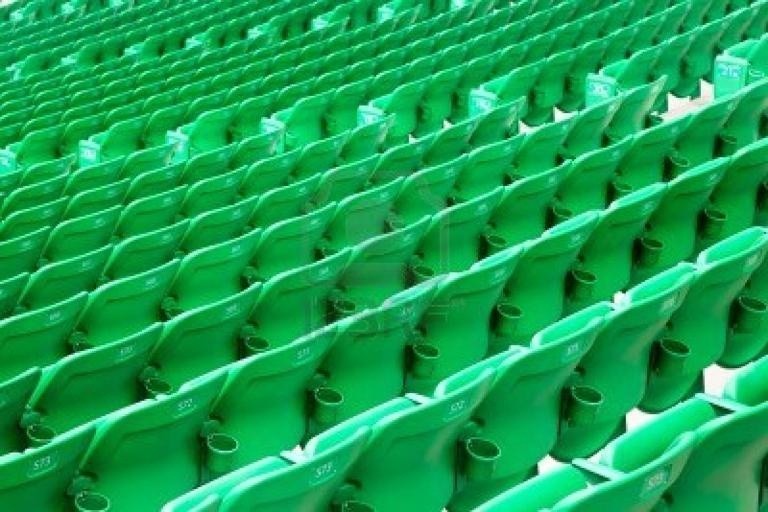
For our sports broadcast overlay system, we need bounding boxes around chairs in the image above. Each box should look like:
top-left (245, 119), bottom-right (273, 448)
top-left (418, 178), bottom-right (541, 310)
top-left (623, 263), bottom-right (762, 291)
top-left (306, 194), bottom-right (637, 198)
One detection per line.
top-left (1, 0), bottom-right (768, 512)
